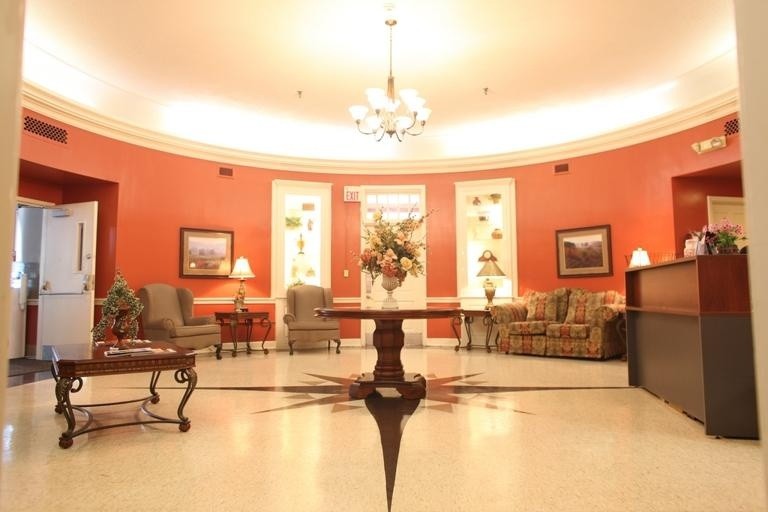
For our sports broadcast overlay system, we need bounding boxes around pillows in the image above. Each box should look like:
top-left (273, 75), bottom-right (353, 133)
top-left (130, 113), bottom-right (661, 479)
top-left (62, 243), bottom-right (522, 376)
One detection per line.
top-left (526, 295), bottom-right (558, 321)
top-left (564, 293), bottom-right (601, 324)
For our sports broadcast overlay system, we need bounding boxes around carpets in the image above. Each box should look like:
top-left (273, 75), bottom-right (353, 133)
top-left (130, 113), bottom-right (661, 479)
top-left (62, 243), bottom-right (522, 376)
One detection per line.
top-left (6, 357), bottom-right (52, 377)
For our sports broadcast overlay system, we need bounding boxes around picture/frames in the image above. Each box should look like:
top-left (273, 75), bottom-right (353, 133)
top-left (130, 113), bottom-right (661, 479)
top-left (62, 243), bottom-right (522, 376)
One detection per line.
top-left (555, 224), bottom-right (613, 279)
top-left (179, 227), bottom-right (235, 281)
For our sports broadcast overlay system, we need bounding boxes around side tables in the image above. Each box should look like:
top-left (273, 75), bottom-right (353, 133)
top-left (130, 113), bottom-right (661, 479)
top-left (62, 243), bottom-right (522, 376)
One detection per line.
top-left (451, 308), bottom-right (500, 354)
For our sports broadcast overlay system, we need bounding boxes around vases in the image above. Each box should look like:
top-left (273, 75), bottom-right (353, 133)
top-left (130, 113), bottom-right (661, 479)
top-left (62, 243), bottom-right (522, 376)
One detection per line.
top-left (717, 244), bottom-right (738, 255)
top-left (380, 273), bottom-right (397, 307)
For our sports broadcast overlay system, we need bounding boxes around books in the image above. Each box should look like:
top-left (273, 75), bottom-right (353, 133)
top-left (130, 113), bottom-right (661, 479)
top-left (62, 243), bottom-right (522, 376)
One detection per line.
top-left (130, 347), bottom-right (176, 357)
top-left (104, 348), bottom-right (151, 356)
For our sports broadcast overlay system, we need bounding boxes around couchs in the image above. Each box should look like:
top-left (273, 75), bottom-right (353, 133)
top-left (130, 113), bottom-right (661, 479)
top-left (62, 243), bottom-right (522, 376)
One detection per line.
top-left (283, 285), bottom-right (341, 355)
top-left (490, 287), bottom-right (627, 362)
top-left (137, 284), bottom-right (222, 361)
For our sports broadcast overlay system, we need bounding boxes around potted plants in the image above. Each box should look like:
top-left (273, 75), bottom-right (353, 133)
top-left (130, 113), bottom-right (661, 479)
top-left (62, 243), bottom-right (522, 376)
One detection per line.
top-left (90, 271), bottom-right (144, 349)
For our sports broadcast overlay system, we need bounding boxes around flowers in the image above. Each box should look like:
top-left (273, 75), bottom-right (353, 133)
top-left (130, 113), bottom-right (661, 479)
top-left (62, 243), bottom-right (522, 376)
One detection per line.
top-left (358, 201), bottom-right (436, 288)
top-left (702, 218), bottom-right (743, 246)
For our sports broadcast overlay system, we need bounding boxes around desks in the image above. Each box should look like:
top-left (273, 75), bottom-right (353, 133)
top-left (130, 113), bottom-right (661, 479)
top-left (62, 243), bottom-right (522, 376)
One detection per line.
top-left (51, 340), bottom-right (197, 448)
top-left (313, 308), bottom-right (462, 399)
top-left (215, 311), bottom-right (271, 359)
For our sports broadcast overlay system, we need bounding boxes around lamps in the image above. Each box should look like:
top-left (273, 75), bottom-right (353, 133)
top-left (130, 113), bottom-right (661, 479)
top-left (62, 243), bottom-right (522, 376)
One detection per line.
top-left (691, 135), bottom-right (727, 154)
top-left (477, 259), bottom-right (504, 310)
top-left (229, 256), bottom-right (256, 311)
top-left (347, 18), bottom-right (432, 142)
